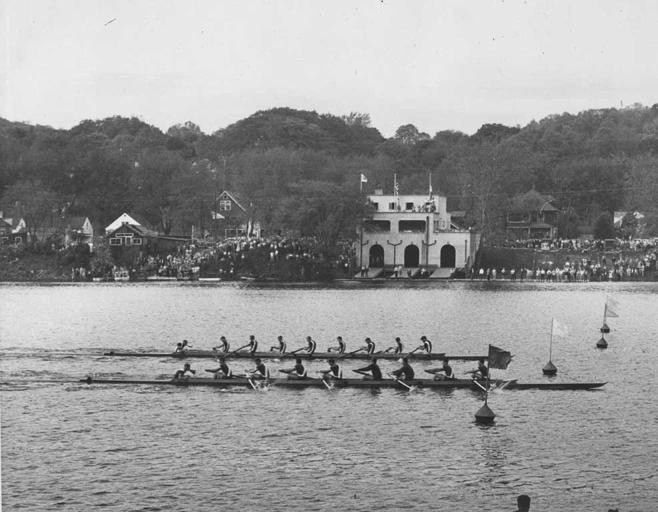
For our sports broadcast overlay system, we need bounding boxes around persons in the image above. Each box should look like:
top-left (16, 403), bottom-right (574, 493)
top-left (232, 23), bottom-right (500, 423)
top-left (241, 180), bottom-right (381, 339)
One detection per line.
top-left (174, 363), bottom-right (196, 378)
top-left (385, 356), bottom-right (413, 379)
top-left (270, 335), bottom-right (285, 354)
top-left (205, 358), bottom-right (230, 377)
top-left (351, 356), bottom-right (382, 380)
top-left (462, 358), bottom-right (490, 381)
top-left (71, 202), bottom-right (658, 284)
top-left (212, 335), bottom-right (230, 353)
top-left (329, 336), bottom-right (346, 352)
top-left (418, 335), bottom-right (432, 353)
top-left (243, 358), bottom-right (269, 378)
top-left (234, 334), bottom-right (258, 353)
top-left (304, 336), bottom-right (317, 354)
top-left (173, 339), bottom-right (194, 355)
top-left (390, 336), bottom-right (403, 354)
top-left (275, 357), bottom-right (309, 380)
top-left (315, 358), bottom-right (342, 380)
top-left (425, 358), bottom-right (455, 379)
top-left (362, 335), bottom-right (375, 354)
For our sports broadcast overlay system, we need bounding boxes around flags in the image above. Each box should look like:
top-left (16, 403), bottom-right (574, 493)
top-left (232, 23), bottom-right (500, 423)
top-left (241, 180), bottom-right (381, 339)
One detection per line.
top-left (605, 304), bottom-right (620, 318)
top-left (607, 296), bottom-right (618, 310)
top-left (429, 174), bottom-right (432, 201)
top-left (488, 345), bottom-right (514, 370)
top-left (394, 173), bottom-right (399, 195)
top-left (361, 173), bottom-right (368, 183)
top-left (551, 318), bottom-right (568, 337)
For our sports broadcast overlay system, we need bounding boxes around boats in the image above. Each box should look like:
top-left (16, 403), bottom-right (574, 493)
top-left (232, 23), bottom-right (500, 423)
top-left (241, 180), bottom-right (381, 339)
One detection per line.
top-left (100, 350), bottom-right (515, 362)
top-left (81, 375), bottom-right (609, 391)
